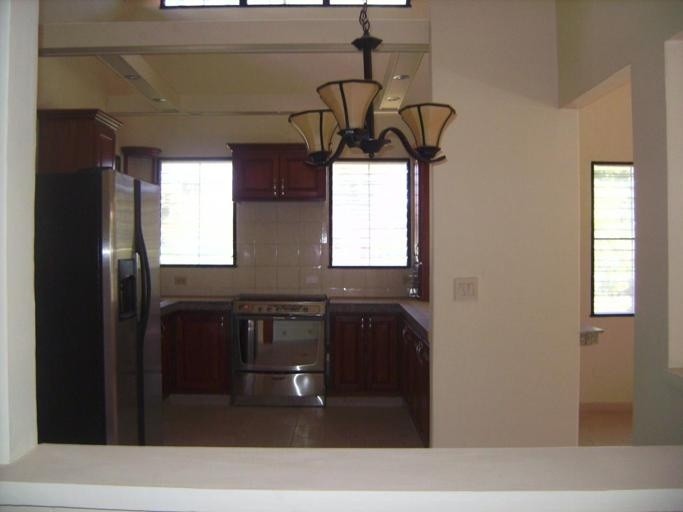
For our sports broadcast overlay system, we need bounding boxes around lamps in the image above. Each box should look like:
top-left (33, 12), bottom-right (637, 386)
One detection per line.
top-left (286, 6), bottom-right (455, 162)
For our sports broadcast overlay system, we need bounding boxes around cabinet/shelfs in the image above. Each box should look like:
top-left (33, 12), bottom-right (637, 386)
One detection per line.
top-left (332, 314), bottom-right (398, 395)
top-left (158, 311), bottom-right (229, 398)
top-left (396, 318), bottom-right (429, 447)
top-left (413, 160), bottom-right (429, 301)
top-left (224, 143), bottom-right (326, 200)
top-left (34, 109), bottom-right (123, 167)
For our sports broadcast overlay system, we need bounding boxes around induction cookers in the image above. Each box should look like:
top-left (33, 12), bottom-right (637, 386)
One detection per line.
top-left (232, 292), bottom-right (328, 315)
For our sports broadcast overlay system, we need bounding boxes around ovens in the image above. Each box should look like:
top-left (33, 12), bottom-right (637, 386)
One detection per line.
top-left (230, 313), bottom-right (325, 407)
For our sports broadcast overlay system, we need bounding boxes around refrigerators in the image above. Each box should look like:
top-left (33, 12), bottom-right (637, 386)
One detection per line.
top-left (35, 168), bottom-right (163, 445)
